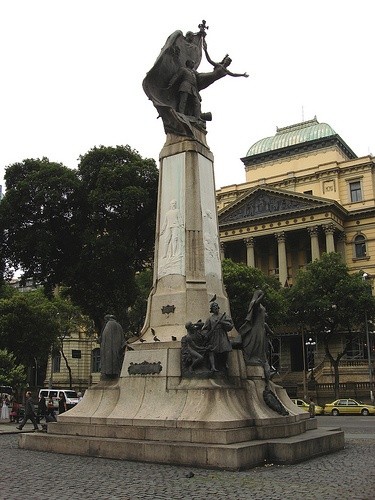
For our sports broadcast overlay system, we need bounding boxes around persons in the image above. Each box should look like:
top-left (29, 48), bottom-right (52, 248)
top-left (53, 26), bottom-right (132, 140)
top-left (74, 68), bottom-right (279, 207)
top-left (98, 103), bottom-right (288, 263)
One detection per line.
top-left (77, 392), bottom-right (83, 403)
top-left (239, 290), bottom-right (275, 366)
top-left (186, 294), bottom-right (234, 372)
top-left (36, 392), bottom-right (57, 424)
top-left (96, 314), bottom-right (125, 378)
top-left (308, 397), bottom-right (315, 418)
top-left (170, 20), bottom-right (250, 123)
top-left (16, 391), bottom-right (39, 431)
top-left (59, 392), bottom-right (67, 414)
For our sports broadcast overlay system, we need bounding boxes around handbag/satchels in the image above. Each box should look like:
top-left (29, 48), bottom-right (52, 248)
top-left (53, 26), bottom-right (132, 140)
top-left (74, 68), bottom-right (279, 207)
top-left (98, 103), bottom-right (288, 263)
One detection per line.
top-left (310, 412), bottom-right (314, 418)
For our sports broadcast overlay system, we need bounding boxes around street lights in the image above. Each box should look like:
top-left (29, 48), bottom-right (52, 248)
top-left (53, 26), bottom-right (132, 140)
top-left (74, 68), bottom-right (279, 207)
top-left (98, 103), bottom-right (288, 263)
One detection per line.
top-left (305, 338), bottom-right (316, 380)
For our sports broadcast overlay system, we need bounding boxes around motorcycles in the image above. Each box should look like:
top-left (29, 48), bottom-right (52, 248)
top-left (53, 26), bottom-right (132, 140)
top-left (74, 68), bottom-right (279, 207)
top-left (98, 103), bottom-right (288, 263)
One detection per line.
top-left (8, 406), bottom-right (46, 423)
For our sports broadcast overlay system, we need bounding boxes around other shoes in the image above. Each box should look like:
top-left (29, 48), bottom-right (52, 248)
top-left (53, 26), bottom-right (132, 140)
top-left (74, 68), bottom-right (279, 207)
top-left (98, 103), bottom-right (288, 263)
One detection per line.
top-left (32, 428), bottom-right (37, 430)
top-left (16, 427), bottom-right (22, 430)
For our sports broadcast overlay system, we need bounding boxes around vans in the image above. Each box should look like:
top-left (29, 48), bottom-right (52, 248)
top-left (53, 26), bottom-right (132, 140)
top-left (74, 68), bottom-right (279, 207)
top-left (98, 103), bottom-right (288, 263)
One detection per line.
top-left (38, 389), bottom-right (81, 411)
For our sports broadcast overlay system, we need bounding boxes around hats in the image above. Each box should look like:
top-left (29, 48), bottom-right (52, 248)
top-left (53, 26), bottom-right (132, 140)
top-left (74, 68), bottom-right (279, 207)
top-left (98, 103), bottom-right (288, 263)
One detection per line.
top-left (27, 391), bottom-right (32, 394)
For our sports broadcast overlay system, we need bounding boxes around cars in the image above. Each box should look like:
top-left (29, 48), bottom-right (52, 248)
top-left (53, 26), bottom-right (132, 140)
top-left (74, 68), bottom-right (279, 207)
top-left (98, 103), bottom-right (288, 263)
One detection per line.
top-left (322, 399), bottom-right (375, 417)
top-left (292, 399), bottom-right (323, 416)
